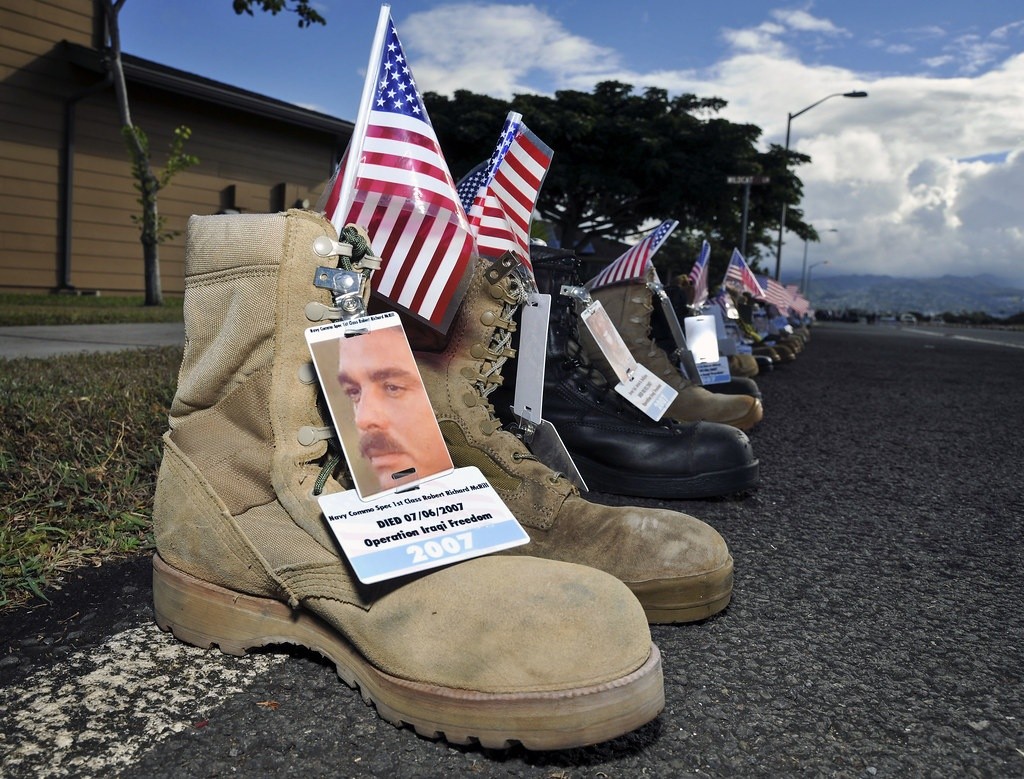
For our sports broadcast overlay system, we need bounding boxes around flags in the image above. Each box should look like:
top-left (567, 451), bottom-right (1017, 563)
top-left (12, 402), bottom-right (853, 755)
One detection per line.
top-left (688, 239), bottom-right (711, 309)
top-left (721, 248), bottom-right (816, 320)
top-left (455, 110), bottom-right (556, 268)
top-left (588, 218), bottom-right (680, 292)
top-left (314, 4), bottom-right (480, 337)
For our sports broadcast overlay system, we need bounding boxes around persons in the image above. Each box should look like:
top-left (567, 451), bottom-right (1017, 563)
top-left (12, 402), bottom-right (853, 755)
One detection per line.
top-left (337, 325), bottom-right (454, 489)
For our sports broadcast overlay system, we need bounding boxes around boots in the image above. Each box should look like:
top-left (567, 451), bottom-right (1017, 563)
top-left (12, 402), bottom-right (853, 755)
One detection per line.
top-left (486, 258), bottom-right (761, 499)
top-left (368, 248), bottom-right (734, 626)
top-left (573, 278), bottom-right (766, 430)
top-left (151, 210), bottom-right (665, 746)
top-left (653, 287), bottom-right (813, 395)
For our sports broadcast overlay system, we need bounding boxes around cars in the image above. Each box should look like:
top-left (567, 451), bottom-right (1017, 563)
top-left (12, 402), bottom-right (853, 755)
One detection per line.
top-left (899, 313), bottom-right (918, 324)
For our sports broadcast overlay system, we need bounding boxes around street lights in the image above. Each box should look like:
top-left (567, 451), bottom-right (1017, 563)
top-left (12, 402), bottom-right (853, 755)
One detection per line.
top-left (775, 91), bottom-right (869, 283)
top-left (805, 260), bottom-right (830, 302)
top-left (800, 228), bottom-right (838, 294)
